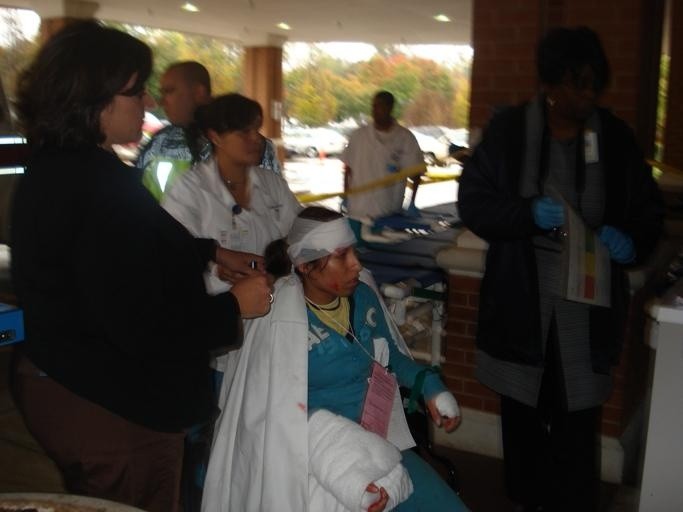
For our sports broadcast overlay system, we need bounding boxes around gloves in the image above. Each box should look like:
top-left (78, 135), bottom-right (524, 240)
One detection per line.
top-left (533, 196), bottom-right (565, 230)
top-left (599, 226), bottom-right (636, 265)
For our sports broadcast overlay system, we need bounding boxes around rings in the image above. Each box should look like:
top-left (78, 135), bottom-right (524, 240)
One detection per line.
top-left (269, 293), bottom-right (274, 303)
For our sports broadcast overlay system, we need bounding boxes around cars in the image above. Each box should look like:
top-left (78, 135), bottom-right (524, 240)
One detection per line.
top-left (281, 116), bottom-right (471, 168)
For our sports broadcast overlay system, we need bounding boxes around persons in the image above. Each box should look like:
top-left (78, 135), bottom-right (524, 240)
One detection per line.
top-left (200, 206), bottom-right (470, 512)
top-left (9, 19), bottom-right (275, 433)
top-left (457, 26), bottom-right (662, 512)
top-left (135, 60), bottom-right (282, 177)
top-left (160, 91), bottom-right (306, 293)
top-left (339, 91), bottom-right (427, 243)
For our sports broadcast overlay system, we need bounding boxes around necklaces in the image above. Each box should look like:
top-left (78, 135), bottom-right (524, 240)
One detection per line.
top-left (221, 178), bottom-right (246, 191)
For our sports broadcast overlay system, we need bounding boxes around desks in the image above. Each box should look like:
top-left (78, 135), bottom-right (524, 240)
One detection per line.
top-left (352, 200), bottom-right (461, 372)
top-left (637, 297), bottom-right (683, 511)
